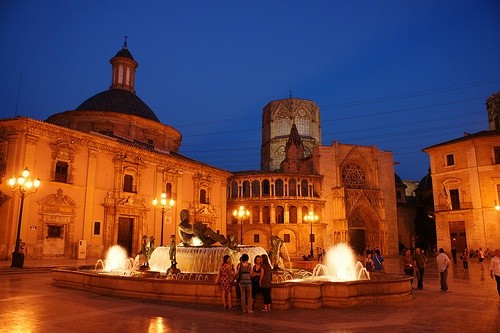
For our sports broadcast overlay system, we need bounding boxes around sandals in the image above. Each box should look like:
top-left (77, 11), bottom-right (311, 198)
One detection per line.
top-left (268, 308), bottom-right (271, 312)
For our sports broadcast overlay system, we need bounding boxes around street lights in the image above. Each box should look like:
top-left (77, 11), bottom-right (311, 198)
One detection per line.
top-left (151, 192), bottom-right (175, 247)
top-left (7, 167), bottom-right (41, 266)
top-left (233, 206), bottom-right (250, 246)
top-left (303, 211), bottom-right (319, 257)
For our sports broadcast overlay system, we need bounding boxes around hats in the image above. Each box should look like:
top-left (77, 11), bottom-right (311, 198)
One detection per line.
top-left (366, 249), bottom-right (372, 254)
top-left (374, 250), bottom-right (380, 255)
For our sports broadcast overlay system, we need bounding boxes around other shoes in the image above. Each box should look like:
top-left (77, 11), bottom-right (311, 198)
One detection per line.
top-left (235, 302), bottom-right (240, 306)
top-left (441, 287), bottom-right (448, 291)
top-left (411, 286), bottom-right (415, 290)
top-left (256, 306), bottom-right (263, 309)
top-left (247, 309), bottom-right (255, 314)
top-left (242, 308), bottom-right (248, 312)
top-left (262, 309), bottom-right (267, 312)
top-left (418, 286), bottom-right (424, 290)
top-left (224, 305), bottom-right (229, 308)
top-left (229, 305), bottom-right (236, 309)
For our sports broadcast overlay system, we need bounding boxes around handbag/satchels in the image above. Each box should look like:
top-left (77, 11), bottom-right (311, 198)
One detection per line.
top-left (460, 255), bottom-right (464, 260)
top-left (237, 275), bottom-right (241, 282)
top-left (217, 272), bottom-right (221, 285)
top-left (381, 262), bottom-right (385, 271)
top-left (478, 258), bottom-right (484, 262)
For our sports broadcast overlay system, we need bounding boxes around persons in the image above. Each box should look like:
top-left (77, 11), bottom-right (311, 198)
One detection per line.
top-left (148, 234), bottom-right (156, 252)
top-left (490, 249), bottom-right (500, 297)
top-left (402, 248), bottom-right (415, 290)
top-left (435, 248), bottom-right (452, 293)
top-left (318, 247), bottom-right (326, 262)
top-left (139, 235), bottom-right (150, 267)
top-left (420, 246), bottom-right (438, 260)
top-left (477, 247), bottom-right (485, 270)
top-left (470, 246), bottom-right (500, 261)
top-left (450, 245), bottom-right (458, 264)
top-left (177, 209), bottom-right (241, 253)
top-left (413, 246), bottom-right (428, 290)
top-left (460, 247), bottom-right (469, 270)
top-left (215, 254), bottom-right (276, 315)
top-left (168, 234), bottom-right (178, 267)
top-left (363, 244), bottom-right (384, 273)
